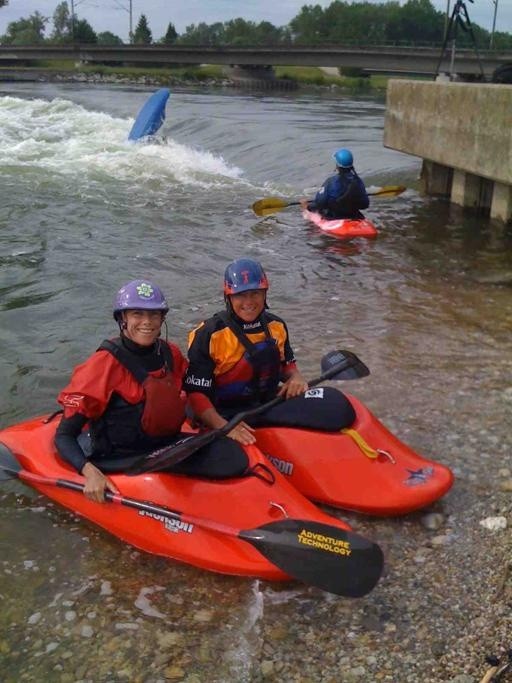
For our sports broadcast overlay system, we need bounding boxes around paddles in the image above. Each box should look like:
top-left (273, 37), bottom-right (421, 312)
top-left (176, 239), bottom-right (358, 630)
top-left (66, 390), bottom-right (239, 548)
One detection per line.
top-left (253, 186), bottom-right (407, 216)
top-left (143, 350), bottom-right (370, 472)
top-left (0, 464), bottom-right (384, 598)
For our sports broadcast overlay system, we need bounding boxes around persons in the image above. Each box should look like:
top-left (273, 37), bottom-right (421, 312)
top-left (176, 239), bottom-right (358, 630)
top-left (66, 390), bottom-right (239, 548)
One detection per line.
top-left (297, 147), bottom-right (369, 221)
top-left (52, 279), bottom-right (190, 506)
top-left (181, 257), bottom-right (309, 448)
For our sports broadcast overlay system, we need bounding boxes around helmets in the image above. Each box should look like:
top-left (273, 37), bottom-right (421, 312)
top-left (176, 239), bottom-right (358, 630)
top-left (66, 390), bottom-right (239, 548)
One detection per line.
top-left (223, 259), bottom-right (269, 298)
top-left (333, 149), bottom-right (353, 169)
top-left (113, 279), bottom-right (168, 323)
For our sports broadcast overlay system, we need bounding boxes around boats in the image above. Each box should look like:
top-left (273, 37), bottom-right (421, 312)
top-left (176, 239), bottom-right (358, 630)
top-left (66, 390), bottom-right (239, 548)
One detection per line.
top-left (1, 409), bottom-right (353, 583)
top-left (249, 386), bottom-right (456, 517)
top-left (301, 203), bottom-right (378, 239)
top-left (128, 87), bottom-right (172, 139)
